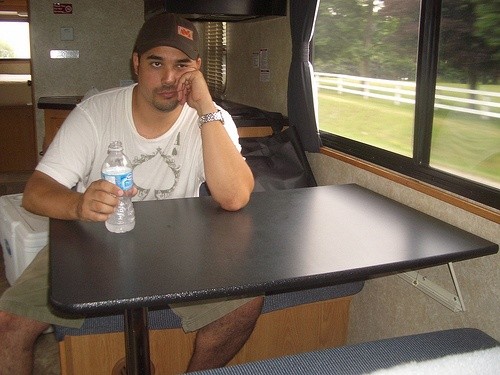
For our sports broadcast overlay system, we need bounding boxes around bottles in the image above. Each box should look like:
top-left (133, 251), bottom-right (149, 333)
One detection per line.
top-left (100, 141), bottom-right (136, 234)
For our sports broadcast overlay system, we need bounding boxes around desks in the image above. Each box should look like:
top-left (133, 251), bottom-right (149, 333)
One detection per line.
top-left (47, 183), bottom-right (500, 375)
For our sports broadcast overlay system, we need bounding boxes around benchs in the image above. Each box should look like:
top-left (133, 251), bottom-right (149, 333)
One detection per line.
top-left (48, 120), bottom-right (499, 375)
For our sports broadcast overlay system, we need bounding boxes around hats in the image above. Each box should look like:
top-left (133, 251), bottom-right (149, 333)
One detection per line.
top-left (134, 15), bottom-right (200, 61)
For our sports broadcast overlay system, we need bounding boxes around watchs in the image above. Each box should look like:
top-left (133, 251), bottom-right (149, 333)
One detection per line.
top-left (197, 108), bottom-right (225, 127)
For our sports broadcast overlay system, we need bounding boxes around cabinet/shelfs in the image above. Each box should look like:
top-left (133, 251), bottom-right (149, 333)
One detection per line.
top-left (37, 96), bottom-right (289, 154)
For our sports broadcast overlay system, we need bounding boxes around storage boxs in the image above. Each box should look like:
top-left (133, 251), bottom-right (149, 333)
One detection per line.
top-left (0, 193), bottom-right (48, 287)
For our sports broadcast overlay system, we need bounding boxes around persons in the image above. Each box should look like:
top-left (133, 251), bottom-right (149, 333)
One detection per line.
top-left (0, 12), bottom-right (265, 375)
